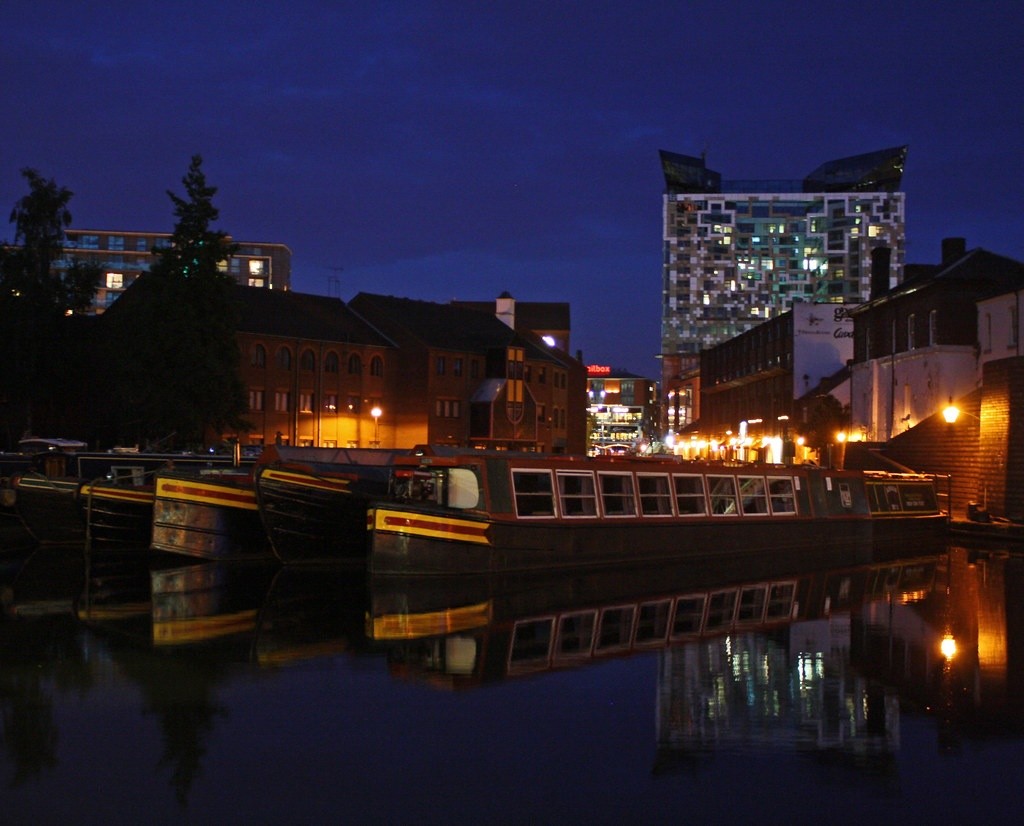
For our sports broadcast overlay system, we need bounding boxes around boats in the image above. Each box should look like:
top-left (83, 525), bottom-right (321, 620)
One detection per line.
top-left (1, 539), bottom-right (369, 675)
top-left (347, 554), bottom-right (950, 689)
top-left (0, 435), bottom-right (953, 652)
